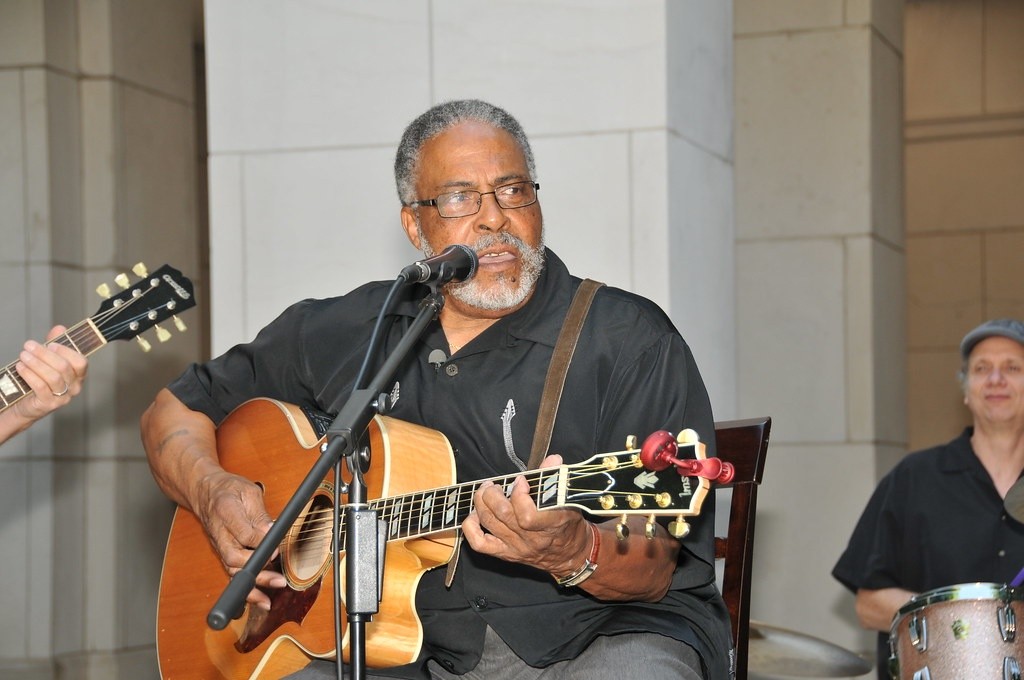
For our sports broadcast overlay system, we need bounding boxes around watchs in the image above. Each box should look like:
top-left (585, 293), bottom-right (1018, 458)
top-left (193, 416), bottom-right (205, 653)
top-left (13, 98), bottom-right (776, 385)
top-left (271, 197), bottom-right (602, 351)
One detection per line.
top-left (549, 523), bottom-right (601, 587)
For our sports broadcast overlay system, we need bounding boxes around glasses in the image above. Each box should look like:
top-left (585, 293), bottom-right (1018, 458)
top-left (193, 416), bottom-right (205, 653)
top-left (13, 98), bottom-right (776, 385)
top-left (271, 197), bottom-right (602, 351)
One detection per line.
top-left (412, 181), bottom-right (540, 218)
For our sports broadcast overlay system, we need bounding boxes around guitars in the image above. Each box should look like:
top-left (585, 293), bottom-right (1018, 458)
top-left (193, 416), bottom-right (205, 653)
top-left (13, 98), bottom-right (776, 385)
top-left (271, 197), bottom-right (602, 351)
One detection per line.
top-left (0, 260), bottom-right (196, 416)
top-left (156, 395), bottom-right (736, 680)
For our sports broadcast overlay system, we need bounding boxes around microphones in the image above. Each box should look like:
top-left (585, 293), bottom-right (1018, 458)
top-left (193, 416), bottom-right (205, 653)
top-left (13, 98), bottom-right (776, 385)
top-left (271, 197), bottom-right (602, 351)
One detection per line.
top-left (398, 244), bottom-right (480, 285)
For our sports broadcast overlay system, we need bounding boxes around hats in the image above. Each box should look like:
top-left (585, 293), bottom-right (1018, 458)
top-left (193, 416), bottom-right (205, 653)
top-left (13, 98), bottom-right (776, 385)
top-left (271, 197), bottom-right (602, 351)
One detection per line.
top-left (961, 320), bottom-right (1024, 360)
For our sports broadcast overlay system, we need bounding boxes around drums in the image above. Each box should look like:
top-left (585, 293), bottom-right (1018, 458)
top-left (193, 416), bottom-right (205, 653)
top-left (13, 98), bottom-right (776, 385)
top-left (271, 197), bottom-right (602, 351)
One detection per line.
top-left (887, 580), bottom-right (1024, 680)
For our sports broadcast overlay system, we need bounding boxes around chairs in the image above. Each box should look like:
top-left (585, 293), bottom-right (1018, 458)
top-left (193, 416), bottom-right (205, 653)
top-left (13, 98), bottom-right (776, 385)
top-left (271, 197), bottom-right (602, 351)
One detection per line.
top-left (714, 417), bottom-right (772, 680)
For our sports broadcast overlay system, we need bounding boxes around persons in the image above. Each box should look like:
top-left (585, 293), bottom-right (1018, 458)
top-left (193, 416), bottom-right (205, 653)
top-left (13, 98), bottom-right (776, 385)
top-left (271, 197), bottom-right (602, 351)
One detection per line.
top-left (1, 325), bottom-right (89, 447)
top-left (831, 318), bottom-right (1024, 680)
top-left (140, 99), bottom-right (737, 679)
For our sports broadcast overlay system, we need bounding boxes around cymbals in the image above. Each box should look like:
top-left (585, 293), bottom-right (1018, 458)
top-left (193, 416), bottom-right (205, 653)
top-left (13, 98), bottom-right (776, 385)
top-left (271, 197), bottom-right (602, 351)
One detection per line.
top-left (746, 622), bottom-right (873, 680)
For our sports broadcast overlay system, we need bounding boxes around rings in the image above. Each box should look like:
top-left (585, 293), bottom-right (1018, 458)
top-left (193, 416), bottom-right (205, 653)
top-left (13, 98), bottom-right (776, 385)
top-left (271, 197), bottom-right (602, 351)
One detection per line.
top-left (52, 383), bottom-right (68, 396)
